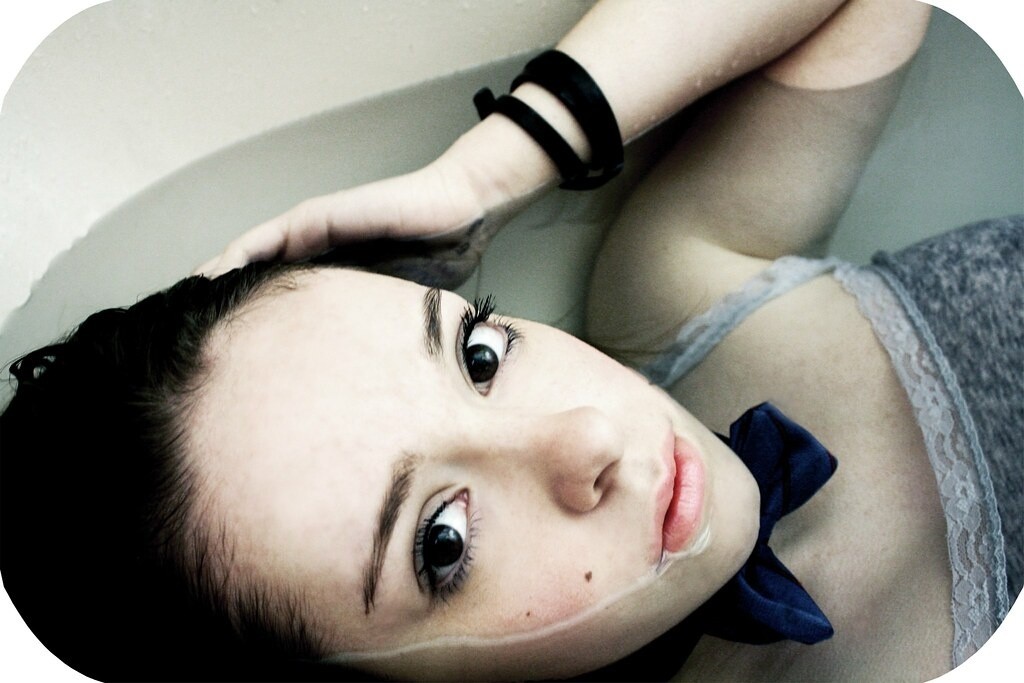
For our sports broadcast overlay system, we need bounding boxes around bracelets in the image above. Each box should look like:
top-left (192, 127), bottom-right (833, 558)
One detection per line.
top-left (472, 49), bottom-right (625, 192)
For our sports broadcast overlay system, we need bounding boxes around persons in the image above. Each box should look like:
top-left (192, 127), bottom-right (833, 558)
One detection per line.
top-left (0, 0), bottom-right (1024, 683)
